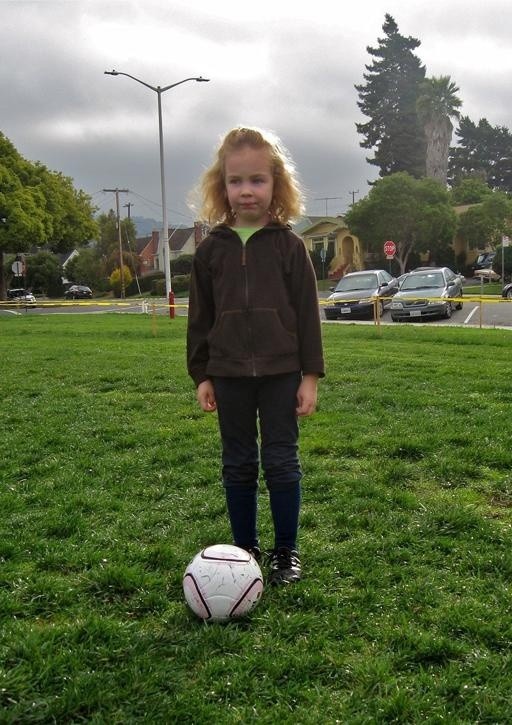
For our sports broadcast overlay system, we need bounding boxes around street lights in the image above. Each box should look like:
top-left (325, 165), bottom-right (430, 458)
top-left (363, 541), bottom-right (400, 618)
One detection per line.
top-left (104, 69), bottom-right (210, 298)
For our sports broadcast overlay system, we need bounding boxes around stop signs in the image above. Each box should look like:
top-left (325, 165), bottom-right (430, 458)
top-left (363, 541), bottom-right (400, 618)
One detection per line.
top-left (384, 240), bottom-right (396, 255)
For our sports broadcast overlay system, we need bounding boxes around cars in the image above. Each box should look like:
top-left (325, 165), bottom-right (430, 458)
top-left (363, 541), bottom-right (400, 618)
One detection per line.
top-left (63, 285), bottom-right (93, 299)
top-left (7, 288), bottom-right (36, 308)
top-left (501, 283), bottom-right (512, 298)
top-left (323, 266), bottom-right (466, 322)
top-left (472, 251), bottom-right (501, 281)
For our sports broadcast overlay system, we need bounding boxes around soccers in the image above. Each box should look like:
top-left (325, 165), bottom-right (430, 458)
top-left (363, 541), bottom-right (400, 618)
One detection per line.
top-left (183, 543), bottom-right (264, 624)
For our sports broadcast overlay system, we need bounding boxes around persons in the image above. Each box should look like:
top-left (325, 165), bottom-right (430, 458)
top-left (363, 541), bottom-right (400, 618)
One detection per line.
top-left (184, 126), bottom-right (327, 589)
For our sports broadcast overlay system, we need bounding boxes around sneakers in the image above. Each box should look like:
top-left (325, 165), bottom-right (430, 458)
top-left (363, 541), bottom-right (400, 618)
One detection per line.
top-left (268, 544), bottom-right (304, 588)
top-left (233, 540), bottom-right (264, 568)
